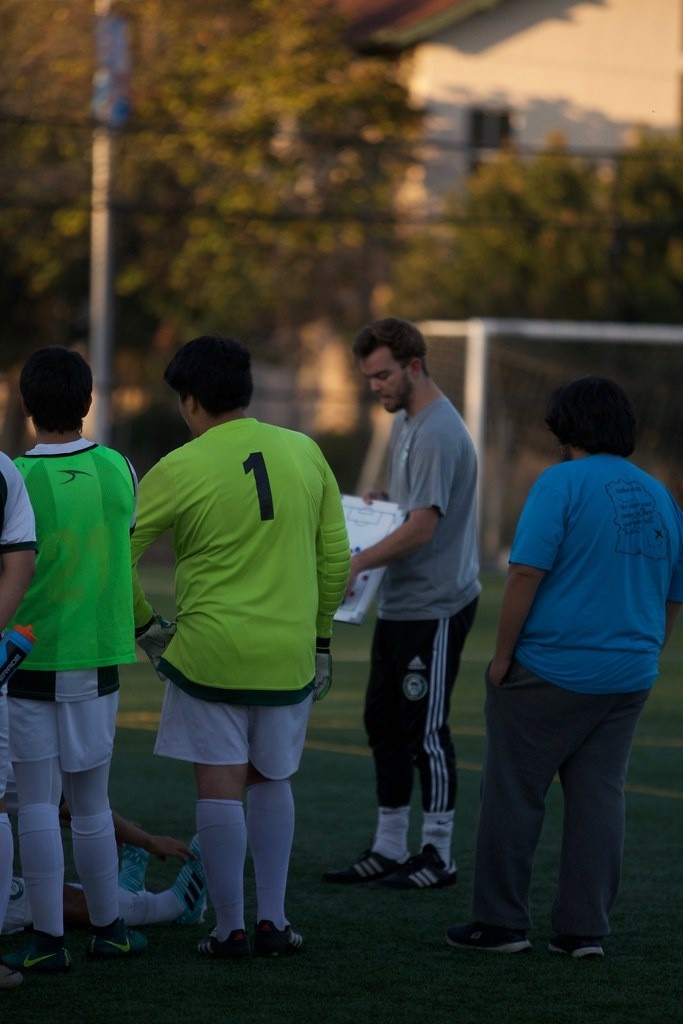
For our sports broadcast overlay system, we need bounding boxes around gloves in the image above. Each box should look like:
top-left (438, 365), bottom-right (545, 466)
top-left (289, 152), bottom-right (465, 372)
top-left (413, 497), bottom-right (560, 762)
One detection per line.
top-left (312, 647), bottom-right (333, 704)
top-left (135, 615), bottom-right (177, 681)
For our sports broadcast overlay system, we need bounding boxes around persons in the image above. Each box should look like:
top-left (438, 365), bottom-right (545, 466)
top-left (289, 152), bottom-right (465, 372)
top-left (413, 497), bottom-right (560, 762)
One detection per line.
top-left (319, 319), bottom-right (481, 891)
top-left (0, 761), bottom-right (211, 936)
top-left (130, 335), bottom-right (351, 957)
top-left (446, 374), bottom-right (683, 957)
top-left (0, 346), bottom-right (136, 977)
top-left (0, 451), bottom-right (38, 988)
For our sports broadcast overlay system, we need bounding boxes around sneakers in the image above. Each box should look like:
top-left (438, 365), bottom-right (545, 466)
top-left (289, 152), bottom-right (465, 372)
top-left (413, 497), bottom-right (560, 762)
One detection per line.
top-left (548, 934), bottom-right (605, 958)
top-left (196, 924), bottom-right (251, 962)
top-left (445, 921), bottom-right (532, 953)
top-left (251, 919), bottom-right (307, 958)
top-left (323, 837), bottom-right (412, 885)
top-left (173, 833), bottom-right (208, 925)
top-left (117, 843), bottom-right (149, 895)
top-left (384, 844), bottom-right (457, 889)
top-left (2, 944), bottom-right (73, 973)
top-left (88, 919), bottom-right (148, 959)
top-left (0, 960), bottom-right (23, 988)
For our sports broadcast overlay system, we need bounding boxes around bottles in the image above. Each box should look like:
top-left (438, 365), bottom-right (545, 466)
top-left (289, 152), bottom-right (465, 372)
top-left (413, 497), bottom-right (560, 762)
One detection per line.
top-left (0, 624), bottom-right (34, 687)
